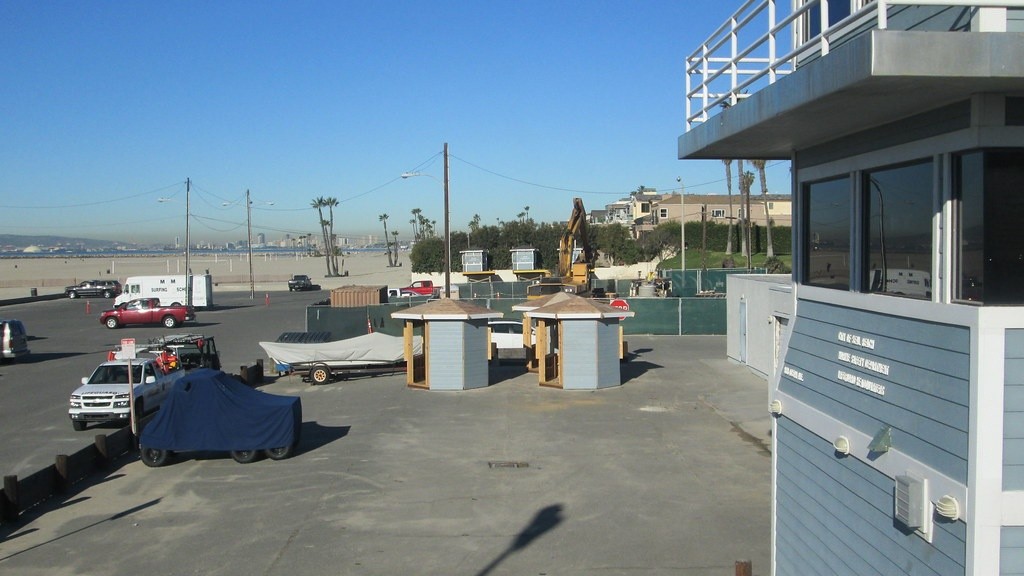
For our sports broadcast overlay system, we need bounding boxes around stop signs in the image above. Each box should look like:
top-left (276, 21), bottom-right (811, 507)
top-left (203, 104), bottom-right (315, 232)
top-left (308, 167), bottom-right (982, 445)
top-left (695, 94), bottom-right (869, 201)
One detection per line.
top-left (610, 299), bottom-right (630, 321)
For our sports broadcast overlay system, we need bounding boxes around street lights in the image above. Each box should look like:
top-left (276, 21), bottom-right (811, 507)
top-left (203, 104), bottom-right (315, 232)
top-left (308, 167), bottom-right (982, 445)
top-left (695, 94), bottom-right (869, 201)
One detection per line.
top-left (401, 171), bottom-right (451, 298)
top-left (222, 201), bottom-right (275, 273)
top-left (676, 175), bottom-right (688, 289)
top-left (158, 196), bottom-right (192, 274)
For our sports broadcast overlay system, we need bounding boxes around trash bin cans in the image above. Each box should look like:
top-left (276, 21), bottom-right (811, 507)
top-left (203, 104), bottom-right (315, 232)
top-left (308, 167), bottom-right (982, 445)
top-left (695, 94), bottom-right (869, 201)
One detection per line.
top-left (15, 264), bottom-right (17, 268)
top-left (107, 269), bottom-right (110, 274)
top-left (30, 288), bottom-right (37, 296)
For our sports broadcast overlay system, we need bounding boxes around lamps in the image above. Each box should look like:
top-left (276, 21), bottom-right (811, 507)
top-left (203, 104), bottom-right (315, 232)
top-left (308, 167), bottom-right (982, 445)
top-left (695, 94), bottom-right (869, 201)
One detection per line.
top-left (832, 436), bottom-right (849, 455)
top-left (936, 495), bottom-right (959, 521)
top-left (769, 399), bottom-right (782, 415)
top-left (867, 425), bottom-right (892, 453)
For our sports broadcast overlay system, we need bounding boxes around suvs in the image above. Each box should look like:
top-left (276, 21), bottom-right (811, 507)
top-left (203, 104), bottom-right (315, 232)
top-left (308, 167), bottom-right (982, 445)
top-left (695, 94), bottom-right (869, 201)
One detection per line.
top-left (64, 280), bottom-right (122, 298)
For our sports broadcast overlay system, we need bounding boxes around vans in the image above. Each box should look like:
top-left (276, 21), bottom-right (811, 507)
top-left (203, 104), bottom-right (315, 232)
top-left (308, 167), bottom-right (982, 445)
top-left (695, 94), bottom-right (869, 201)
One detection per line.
top-left (0, 320), bottom-right (31, 359)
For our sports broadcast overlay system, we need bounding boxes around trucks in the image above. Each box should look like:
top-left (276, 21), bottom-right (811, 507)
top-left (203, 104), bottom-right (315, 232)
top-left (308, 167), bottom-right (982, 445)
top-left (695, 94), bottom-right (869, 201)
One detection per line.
top-left (388, 288), bottom-right (432, 299)
top-left (116, 274), bottom-right (214, 308)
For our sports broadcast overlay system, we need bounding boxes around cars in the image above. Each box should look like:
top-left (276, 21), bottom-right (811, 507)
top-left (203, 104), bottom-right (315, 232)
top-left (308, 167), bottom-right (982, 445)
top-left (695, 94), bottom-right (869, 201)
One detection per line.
top-left (402, 290), bottom-right (420, 296)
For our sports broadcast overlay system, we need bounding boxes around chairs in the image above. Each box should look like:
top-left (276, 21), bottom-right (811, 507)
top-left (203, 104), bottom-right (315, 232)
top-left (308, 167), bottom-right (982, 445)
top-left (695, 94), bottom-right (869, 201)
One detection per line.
top-left (108, 367), bottom-right (126, 381)
top-left (133, 367), bottom-right (141, 381)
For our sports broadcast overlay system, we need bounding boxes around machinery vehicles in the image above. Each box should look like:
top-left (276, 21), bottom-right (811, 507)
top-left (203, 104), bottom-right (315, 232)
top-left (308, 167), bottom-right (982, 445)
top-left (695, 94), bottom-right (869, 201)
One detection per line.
top-left (522, 197), bottom-right (595, 299)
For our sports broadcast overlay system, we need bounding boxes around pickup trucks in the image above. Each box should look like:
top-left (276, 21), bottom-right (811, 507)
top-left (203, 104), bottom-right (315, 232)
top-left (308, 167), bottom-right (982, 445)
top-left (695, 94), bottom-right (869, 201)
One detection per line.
top-left (288, 274), bottom-right (312, 292)
top-left (70, 358), bottom-right (186, 430)
top-left (400, 280), bottom-right (443, 296)
top-left (99, 297), bottom-right (187, 329)
